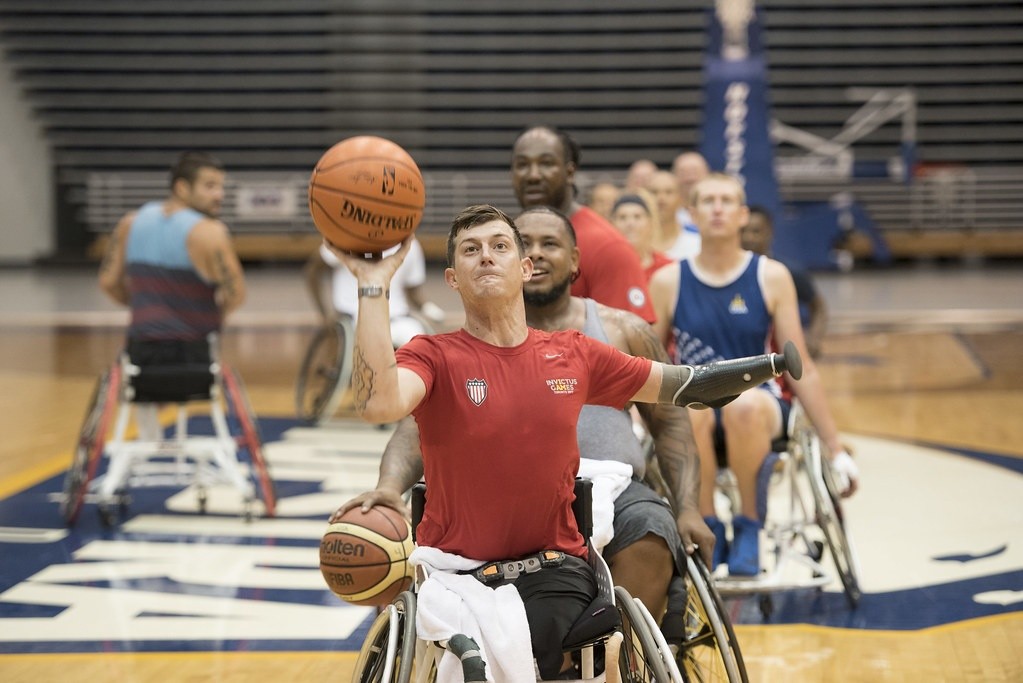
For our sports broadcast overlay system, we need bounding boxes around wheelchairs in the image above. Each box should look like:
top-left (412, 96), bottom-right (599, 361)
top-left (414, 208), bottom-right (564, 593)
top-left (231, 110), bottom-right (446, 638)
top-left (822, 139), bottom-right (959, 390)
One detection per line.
top-left (296, 312), bottom-right (441, 426)
top-left (636, 415), bottom-right (862, 608)
top-left (60, 329), bottom-right (278, 533)
top-left (351, 529), bottom-right (750, 683)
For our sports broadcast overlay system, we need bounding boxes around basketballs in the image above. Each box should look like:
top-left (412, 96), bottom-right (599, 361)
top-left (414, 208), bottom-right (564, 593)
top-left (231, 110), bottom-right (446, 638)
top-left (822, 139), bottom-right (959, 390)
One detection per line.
top-left (318, 504), bottom-right (418, 608)
top-left (306, 133), bottom-right (427, 255)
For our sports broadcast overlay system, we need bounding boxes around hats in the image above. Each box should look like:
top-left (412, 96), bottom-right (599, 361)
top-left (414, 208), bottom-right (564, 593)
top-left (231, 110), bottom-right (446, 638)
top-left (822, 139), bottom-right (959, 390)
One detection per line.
top-left (612, 193), bottom-right (652, 216)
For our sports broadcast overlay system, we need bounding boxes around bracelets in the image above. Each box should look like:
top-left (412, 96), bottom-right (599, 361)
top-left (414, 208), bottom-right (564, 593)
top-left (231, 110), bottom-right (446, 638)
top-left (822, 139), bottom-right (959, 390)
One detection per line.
top-left (357, 287), bottom-right (390, 299)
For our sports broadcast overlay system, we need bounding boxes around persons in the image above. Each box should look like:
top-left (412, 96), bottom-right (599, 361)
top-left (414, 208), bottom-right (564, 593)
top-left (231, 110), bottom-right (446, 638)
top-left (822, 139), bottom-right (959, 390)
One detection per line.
top-left (99, 150), bottom-right (245, 402)
top-left (648, 175), bottom-right (859, 575)
top-left (510, 122), bottom-right (656, 324)
top-left (741, 207), bottom-right (826, 358)
top-left (584, 184), bottom-right (618, 216)
top-left (328, 206), bottom-right (716, 644)
top-left (646, 171), bottom-right (702, 262)
top-left (323, 205), bottom-right (802, 655)
top-left (305, 233), bottom-right (445, 356)
top-left (624, 159), bottom-right (658, 190)
top-left (608, 191), bottom-right (675, 285)
top-left (671, 151), bottom-right (712, 210)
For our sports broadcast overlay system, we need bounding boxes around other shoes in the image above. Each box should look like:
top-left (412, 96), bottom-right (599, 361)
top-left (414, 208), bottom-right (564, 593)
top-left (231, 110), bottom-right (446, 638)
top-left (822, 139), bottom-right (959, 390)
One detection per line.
top-left (728, 517), bottom-right (759, 578)
top-left (704, 516), bottom-right (728, 574)
